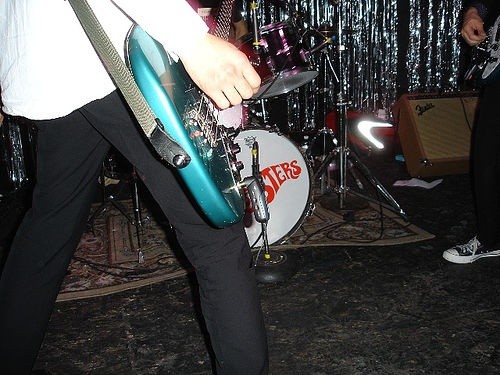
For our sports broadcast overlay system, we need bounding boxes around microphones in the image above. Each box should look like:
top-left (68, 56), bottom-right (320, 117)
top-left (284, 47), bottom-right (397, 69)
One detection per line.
top-left (310, 37), bottom-right (333, 56)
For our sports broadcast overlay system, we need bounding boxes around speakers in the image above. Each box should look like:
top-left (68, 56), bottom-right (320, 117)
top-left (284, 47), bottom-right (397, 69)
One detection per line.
top-left (393, 90), bottom-right (481, 177)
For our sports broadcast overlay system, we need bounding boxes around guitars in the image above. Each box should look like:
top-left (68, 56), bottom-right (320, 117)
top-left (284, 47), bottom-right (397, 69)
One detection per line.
top-left (473, 7), bottom-right (500, 83)
top-left (125, 0), bottom-right (245, 228)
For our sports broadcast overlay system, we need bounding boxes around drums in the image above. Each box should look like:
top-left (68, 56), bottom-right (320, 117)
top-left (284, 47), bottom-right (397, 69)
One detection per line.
top-left (227, 126), bottom-right (315, 247)
top-left (232, 21), bottom-right (319, 100)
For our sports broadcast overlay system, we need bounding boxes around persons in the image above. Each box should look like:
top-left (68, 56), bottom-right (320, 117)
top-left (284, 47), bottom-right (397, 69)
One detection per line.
top-left (0, 0), bottom-right (268, 375)
top-left (442, 0), bottom-right (500, 264)
top-left (188, 0), bottom-right (248, 48)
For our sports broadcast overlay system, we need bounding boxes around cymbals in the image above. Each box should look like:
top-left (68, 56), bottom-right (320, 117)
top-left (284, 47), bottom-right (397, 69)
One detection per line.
top-left (303, 27), bottom-right (359, 36)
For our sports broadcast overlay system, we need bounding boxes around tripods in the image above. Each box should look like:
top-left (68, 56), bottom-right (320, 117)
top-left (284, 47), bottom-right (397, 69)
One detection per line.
top-left (306, 0), bottom-right (409, 224)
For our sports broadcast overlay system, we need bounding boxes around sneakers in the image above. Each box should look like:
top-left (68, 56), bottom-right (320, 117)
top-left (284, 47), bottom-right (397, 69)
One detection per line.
top-left (442, 234), bottom-right (500, 263)
